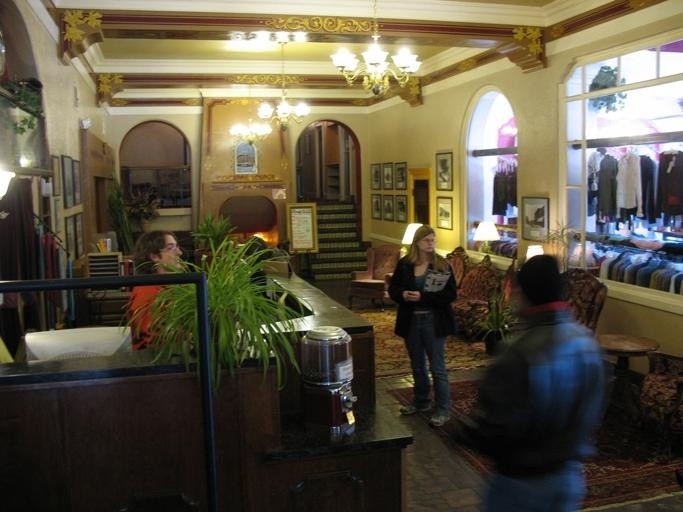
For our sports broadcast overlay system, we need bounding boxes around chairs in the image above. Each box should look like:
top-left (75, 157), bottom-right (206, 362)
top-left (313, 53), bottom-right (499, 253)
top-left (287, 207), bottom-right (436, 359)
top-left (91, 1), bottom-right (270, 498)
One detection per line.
top-left (449, 254), bottom-right (501, 336)
top-left (560, 266), bottom-right (608, 331)
top-left (639, 349), bottom-right (682, 432)
top-left (441, 245), bottom-right (469, 288)
top-left (347, 243), bottom-right (404, 312)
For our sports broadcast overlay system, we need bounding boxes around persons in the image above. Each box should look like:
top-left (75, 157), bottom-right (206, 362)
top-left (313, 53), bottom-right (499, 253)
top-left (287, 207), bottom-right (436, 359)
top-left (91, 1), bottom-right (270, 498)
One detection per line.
top-left (388, 226), bottom-right (457, 428)
top-left (468, 255), bottom-right (607, 512)
top-left (125, 230), bottom-right (194, 352)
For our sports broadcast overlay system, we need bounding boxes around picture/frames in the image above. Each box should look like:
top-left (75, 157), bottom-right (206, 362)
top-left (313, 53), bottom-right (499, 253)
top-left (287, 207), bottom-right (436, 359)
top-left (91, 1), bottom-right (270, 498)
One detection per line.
top-left (435, 195), bottom-right (453, 231)
top-left (434, 151), bottom-right (453, 192)
top-left (519, 195), bottom-right (550, 244)
top-left (369, 160), bottom-right (408, 224)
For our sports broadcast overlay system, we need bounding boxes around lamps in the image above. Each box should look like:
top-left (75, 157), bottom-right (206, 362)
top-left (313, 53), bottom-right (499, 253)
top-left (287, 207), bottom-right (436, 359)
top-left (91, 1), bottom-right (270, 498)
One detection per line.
top-left (472, 221), bottom-right (501, 253)
top-left (327, 1), bottom-right (422, 95)
top-left (226, 120), bottom-right (272, 146)
top-left (401, 222), bottom-right (424, 246)
top-left (256, 39), bottom-right (312, 131)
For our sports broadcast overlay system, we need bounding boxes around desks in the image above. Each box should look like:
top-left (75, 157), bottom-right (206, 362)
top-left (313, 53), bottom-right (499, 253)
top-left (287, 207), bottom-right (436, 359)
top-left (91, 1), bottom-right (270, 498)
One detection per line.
top-left (593, 334), bottom-right (659, 418)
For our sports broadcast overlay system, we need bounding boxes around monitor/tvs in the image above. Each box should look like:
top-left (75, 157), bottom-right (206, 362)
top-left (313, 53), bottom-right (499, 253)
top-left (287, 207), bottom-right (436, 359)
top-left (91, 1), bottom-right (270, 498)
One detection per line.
top-left (0, 86), bottom-right (55, 178)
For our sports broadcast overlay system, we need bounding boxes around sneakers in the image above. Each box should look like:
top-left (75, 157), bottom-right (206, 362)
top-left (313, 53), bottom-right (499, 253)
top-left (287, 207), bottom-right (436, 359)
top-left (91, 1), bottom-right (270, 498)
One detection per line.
top-left (399, 400), bottom-right (432, 414)
top-left (429, 410), bottom-right (450, 427)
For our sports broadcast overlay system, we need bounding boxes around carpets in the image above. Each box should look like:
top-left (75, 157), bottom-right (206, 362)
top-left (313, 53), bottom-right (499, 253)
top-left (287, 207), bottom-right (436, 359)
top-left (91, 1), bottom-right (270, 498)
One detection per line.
top-left (355, 306), bottom-right (498, 379)
top-left (385, 359), bottom-right (682, 511)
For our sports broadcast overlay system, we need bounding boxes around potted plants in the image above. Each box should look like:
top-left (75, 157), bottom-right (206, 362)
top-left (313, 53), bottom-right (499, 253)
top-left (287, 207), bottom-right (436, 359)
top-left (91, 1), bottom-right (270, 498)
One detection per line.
top-left (472, 290), bottom-right (521, 355)
top-left (120, 231), bottom-right (315, 395)
